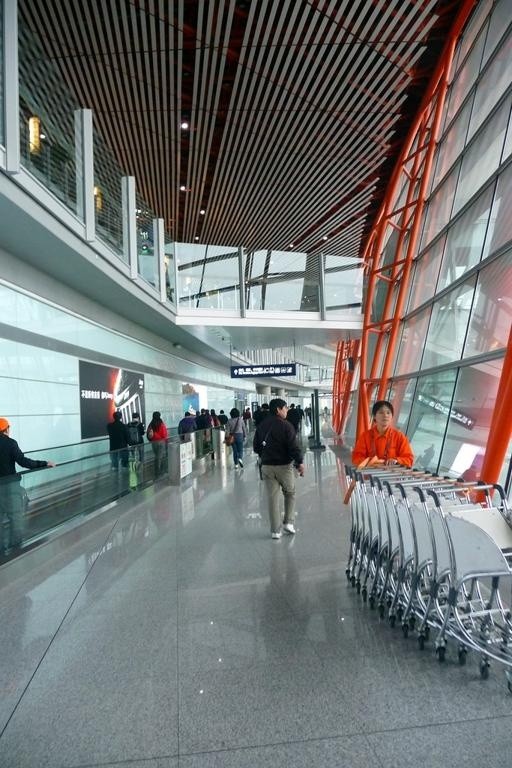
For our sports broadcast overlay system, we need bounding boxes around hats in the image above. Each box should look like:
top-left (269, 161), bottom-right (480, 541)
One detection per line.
top-left (0, 418), bottom-right (9, 431)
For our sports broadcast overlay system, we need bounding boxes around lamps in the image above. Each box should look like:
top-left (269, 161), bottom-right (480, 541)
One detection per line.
top-left (29, 116), bottom-right (42, 154)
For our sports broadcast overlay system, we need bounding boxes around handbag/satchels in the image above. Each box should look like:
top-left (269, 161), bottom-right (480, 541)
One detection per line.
top-left (148, 429), bottom-right (153, 439)
top-left (225, 434), bottom-right (234, 446)
top-left (259, 463), bottom-right (263, 480)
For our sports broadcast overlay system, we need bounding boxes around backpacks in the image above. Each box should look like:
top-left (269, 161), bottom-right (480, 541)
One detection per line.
top-left (127, 422), bottom-right (141, 445)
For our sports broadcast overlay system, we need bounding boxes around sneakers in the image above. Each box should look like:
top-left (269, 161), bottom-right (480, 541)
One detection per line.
top-left (235, 458), bottom-right (243, 469)
top-left (271, 522), bottom-right (295, 539)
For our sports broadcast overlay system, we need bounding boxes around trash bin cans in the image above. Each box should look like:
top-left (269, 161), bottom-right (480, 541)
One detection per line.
top-left (168, 440), bottom-right (192, 479)
top-left (212, 427), bottom-right (226, 458)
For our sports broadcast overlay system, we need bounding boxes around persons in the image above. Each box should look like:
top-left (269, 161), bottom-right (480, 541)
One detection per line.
top-left (106, 409), bottom-right (131, 484)
top-left (250, 396), bottom-right (308, 539)
top-left (125, 411), bottom-right (147, 469)
top-left (0, 416), bottom-right (57, 557)
top-left (177, 401), bottom-right (312, 469)
top-left (145, 408), bottom-right (168, 477)
top-left (350, 398), bottom-right (417, 468)
top-left (419, 443), bottom-right (436, 468)
top-left (455, 464), bottom-right (480, 498)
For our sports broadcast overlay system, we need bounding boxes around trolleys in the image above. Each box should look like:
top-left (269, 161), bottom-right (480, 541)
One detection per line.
top-left (341, 460), bottom-right (511, 691)
